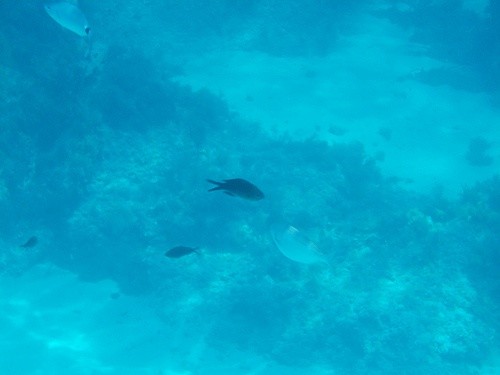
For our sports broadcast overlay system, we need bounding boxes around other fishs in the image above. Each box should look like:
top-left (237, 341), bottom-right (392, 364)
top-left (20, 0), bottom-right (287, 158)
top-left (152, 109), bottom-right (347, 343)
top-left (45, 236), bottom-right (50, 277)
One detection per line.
top-left (42, 1), bottom-right (92, 42)
top-left (165, 246), bottom-right (199, 259)
top-left (20, 236), bottom-right (38, 249)
top-left (269, 223), bottom-right (332, 267)
top-left (205, 178), bottom-right (265, 202)
